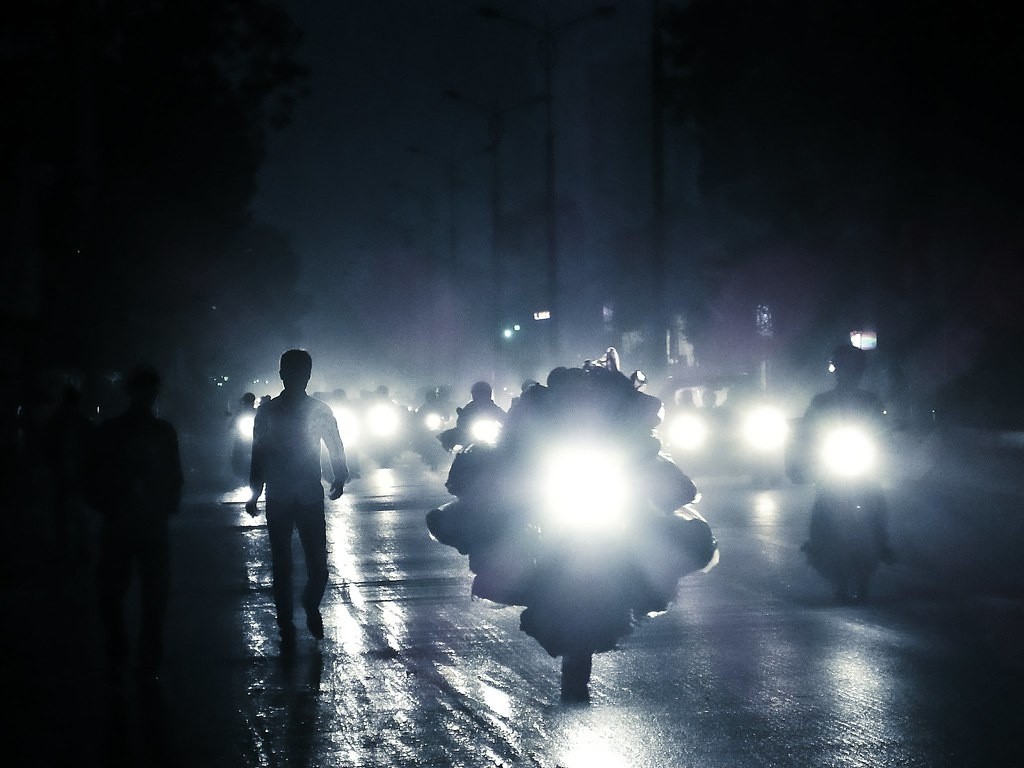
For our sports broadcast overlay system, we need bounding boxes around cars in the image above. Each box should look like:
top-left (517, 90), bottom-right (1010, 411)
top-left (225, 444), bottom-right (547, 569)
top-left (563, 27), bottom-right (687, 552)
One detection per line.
top-left (657, 373), bottom-right (784, 484)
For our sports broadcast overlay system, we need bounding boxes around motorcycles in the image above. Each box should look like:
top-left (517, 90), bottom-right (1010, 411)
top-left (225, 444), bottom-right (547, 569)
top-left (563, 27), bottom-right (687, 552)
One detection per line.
top-left (230, 404), bottom-right (942, 699)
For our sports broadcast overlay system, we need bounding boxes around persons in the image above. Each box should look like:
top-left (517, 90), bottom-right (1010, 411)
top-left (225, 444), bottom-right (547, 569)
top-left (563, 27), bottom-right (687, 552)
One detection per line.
top-left (226, 393), bottom-right (256, 466)
top-left (82, 365), bottom-right (182, 678)
top-left (678, 391), bottom-right (695, 408)
top-left (245, 349), bottom-right (351, 647)
top-left (700, 390), bottom-right (716, 409)
top-left (258, 386), bottom-right (451, 429)
top-left (46, 386), bottom-right (96, 573)
top-left (457, 383), bottom-right (506, 424)
top-left (800, 344), bottom-right (898, 567)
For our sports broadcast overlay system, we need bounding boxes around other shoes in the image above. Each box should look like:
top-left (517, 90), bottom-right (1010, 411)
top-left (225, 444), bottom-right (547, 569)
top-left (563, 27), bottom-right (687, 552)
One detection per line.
top-left (302, 601), bottom-right (324, 639)
top-left (277, 620), bottom-right (297, 633)
